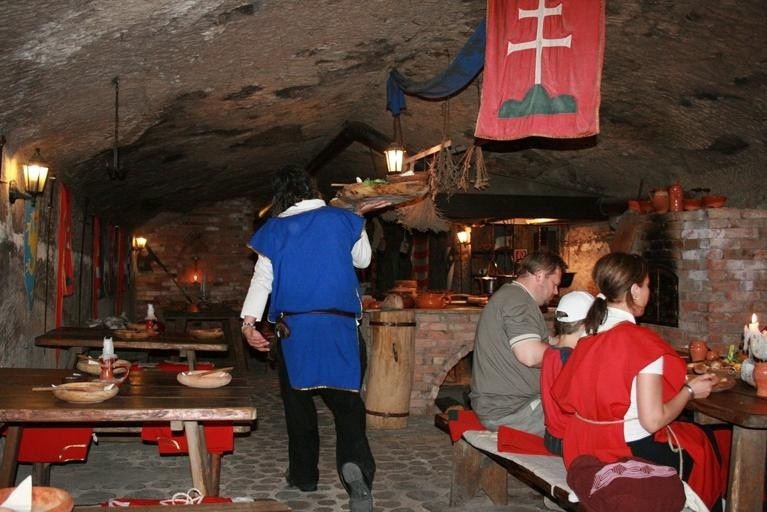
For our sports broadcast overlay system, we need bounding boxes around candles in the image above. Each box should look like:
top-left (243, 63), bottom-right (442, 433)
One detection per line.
top-left (746, 312), bottom-right (759, 330)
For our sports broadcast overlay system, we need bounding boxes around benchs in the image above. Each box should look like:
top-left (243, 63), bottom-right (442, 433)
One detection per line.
top-left (433, 409), bottom-right (580, 511)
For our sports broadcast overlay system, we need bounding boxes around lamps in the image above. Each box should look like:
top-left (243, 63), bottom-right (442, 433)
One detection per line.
top-left (381, 111), bottom-right (411, 173)
top-left (8, 145), bottom-right (52, 207)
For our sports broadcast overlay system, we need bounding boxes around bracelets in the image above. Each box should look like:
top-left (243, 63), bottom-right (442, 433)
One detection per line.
top-left (682, 384), bottom-right (695, 402)
top-left (239, 321), bottom-right (255, 330)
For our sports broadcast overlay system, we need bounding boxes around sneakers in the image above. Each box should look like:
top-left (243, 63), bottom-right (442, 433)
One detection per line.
top-left (342, 462), bottom-right (373, 512)
top-left (286, 467), bottom-right (317, 492)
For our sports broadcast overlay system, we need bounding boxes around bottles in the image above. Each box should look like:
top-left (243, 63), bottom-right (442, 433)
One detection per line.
top-left (144, 302), bottom-right (157, 331)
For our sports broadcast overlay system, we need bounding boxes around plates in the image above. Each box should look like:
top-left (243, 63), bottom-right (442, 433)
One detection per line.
top-left (0, 485), bottom-right (74, 512)
top-left (176, 369), bottom-right (232, 389)
top-left (685, 374), bottom-right (736, 393)
top-left (112, 329), bottom-right (150, 341)
top-left (189, 328), bottom-right (225, 340)
top-left (51, 381), bottom-right (120, 405)
top-left (126, 323), bottom-right (159, 331)
top-left (75, 358), bottom-right (132, 376)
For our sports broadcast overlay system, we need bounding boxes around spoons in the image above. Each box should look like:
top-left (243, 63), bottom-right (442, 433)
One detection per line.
top-left (31, 386), bottom-right (97, 393)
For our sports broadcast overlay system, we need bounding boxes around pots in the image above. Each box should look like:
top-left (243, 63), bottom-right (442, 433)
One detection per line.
top-left (414, 293), bottom-right (452, 308)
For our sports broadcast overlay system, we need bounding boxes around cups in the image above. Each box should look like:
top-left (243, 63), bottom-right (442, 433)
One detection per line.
top-left (752, 362), bottom-right (766, 399)
top-left (688, 340), bottom-right (708, 362)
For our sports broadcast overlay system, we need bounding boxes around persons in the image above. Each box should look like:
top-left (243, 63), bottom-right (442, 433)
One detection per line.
top-left (547, 252), bottom-right (734, 510)
top-left (466, 251), bottom-right (559, 438)
top-left (539, 290), bottom-right (597, 455)
top-left (238, 162), bottom-right (378, 511)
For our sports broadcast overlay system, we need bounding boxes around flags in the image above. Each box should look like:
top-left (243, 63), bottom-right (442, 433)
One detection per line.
top-left (473, 0), bottom-right (607, 144)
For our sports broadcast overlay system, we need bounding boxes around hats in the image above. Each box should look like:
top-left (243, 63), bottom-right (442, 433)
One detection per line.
top-left (555, 291), bottom-right (596, 323)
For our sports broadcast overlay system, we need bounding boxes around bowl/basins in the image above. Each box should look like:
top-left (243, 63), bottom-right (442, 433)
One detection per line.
top-left (627, 181), bottom-right (728, 214)
